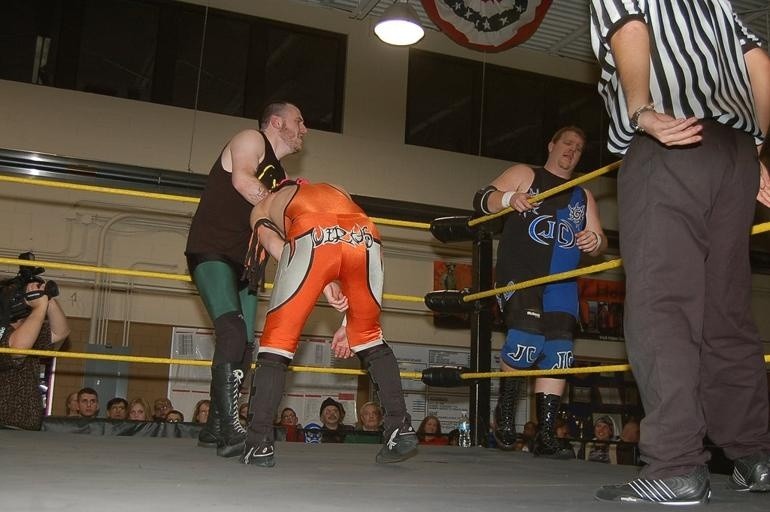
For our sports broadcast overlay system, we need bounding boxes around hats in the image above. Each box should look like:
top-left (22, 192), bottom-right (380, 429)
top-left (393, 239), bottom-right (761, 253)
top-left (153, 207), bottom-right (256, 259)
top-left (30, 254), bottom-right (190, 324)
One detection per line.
top-left (320, 397), bottom-right (340, 416)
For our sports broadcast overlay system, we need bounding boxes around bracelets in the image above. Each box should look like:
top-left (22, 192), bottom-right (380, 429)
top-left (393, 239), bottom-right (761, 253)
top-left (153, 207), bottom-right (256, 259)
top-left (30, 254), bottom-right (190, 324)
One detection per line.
top-left (628, 106), bottom-right (656, 132)
top-left (341, 314), bottom-right (347, 327)
top-left (595, 232), bottom-right (602, 253)
top-left (502, 190), bottom-right (516, 208)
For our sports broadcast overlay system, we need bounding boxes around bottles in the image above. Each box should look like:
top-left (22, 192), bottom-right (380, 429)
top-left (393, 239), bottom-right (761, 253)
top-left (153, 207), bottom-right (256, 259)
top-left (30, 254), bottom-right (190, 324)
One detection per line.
top-left (458, 409), bottom-right (472, 449)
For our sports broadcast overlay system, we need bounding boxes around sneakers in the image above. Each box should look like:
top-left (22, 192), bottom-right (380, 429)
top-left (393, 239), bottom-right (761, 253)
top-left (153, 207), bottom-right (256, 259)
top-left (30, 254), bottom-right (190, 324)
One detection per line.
top-left (240, 437), bottom-right (275, 467)
top-left (376, 435), bottom-right (416, 464)
top-left (727, 452), bottom-right (770, 493)
top-left (594, 463), bottom-right (711, 505)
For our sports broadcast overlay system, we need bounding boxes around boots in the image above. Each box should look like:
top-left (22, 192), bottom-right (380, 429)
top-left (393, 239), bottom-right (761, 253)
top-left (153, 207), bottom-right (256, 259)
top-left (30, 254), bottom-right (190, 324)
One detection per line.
top-left (494, 370), bottom-right (522, 451)
top-left (211, 362), bottom-right (249, 457)
top-left (533, 392), bottom-right (572, 459)
top-left (198, 368), bottom-right (247, 448)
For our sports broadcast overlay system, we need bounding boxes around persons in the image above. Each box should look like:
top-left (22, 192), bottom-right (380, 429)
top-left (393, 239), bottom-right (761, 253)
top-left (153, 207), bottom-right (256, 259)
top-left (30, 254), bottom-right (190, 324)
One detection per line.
top-left (66, 388), bottom-right (384, 431)
top-left (476, 126), bottom-right (609, 458)
top-left (418, 415), bottom-right (620, 464)
top-left (0, 278), bottom-right (72, 429)
top-left (588, 1), bottom-right (769, 506)
top-left (186, 98), bottom-right (307, 456)
top-left (235, 180), bottom-right (418, 469)
